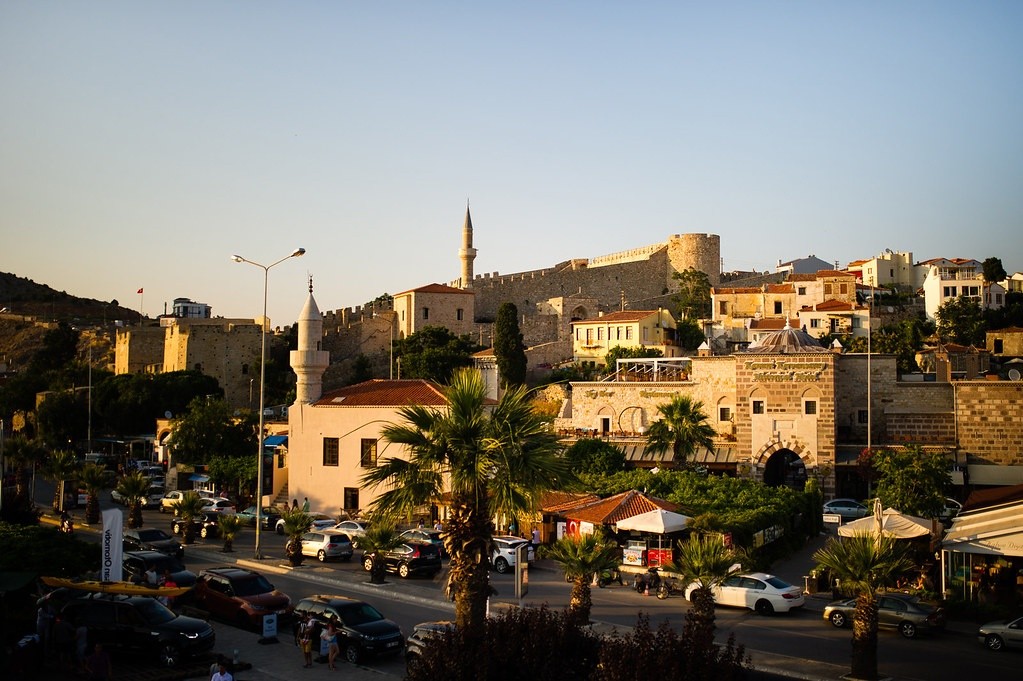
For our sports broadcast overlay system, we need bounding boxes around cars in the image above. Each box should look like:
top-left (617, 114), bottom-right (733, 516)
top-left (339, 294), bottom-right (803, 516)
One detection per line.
top-left (405, 620), bottom-right (461, 681)
top-left (824, 499), bottom-right (872, 520)
top-left (122, 550), bottom-right (200, 589)
top-left (683, 571), bottom-right (805, 617)
top-left (325, 521), bottom-right (372, 550)
top-left (169, 511), bottom-right (228, 539)
top-left (110, 460), bottom-right (165, 508)
top-left (918, 497), bottom-right (963, 517)
top-left (122, 527), bottom-right (185, 558)
top-left (234, 506), bottom-right (282, 530)
top-left (195, 566), bottom-right (294, 628)
top-left (198, 497), bottom-right (237, 515)
top-left (274, 512), bottom-right (336, 535)
top-left (978, 615), bottom-right (1022, 651)
top-left (77, 490), bottom-right (89, 505)
top-left (823, 594), bottom-right (939, 640)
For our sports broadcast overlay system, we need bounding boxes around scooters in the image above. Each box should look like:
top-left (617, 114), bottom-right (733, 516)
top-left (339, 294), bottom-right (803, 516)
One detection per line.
top-left (60, 520), bottom-right (74, 536)
top-left (597, 564), bottom-right (624, 588)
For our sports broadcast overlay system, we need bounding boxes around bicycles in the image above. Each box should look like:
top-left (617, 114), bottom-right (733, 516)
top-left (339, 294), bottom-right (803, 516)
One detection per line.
top-left (654, 575), bottom-right (678, 600)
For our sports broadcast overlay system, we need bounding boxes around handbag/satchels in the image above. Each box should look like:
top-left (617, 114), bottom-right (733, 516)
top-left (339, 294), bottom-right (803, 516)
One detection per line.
top-left (320, 626), bottom-right (336, 642)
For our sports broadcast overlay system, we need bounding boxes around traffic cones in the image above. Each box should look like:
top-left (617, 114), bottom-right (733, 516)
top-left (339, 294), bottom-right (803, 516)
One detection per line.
top-left (641, 583), bottom-right (651, 596)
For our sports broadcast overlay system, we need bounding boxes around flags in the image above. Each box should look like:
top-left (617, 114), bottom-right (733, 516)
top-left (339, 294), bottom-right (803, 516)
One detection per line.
top-left (137, 288), bottom-right (143, 293)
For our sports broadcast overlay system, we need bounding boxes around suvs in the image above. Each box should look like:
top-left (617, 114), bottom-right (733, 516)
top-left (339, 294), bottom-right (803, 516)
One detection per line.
top-left (286, 531), bottom-right (353, 562)
top-left (291, 594), bottom-right (405, 665)
top-left (389, 528), bottom-right (448, 558)
top-left (35, 588), bottom-right (216, 672)
top-left (160, 490), bottom-right (200, 517)
top-left (476, 535), bottom-right (534, 574)
top-left (360, 538), bottom-right (444, 579)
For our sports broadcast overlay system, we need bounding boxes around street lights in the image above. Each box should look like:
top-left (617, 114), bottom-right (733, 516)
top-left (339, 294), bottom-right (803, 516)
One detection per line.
top-left (71, 326), bottom-right (93, 453)
top-left (864, 295), bottom-right (877, 506)
top-left (230, 248), bottom-right (307, 559)
top-left (372, 312), bottom-right (394, 380)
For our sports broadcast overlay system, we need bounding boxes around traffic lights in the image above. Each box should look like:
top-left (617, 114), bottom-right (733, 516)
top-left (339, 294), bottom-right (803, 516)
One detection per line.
top-left (162, 460), bottom-right (169, 472)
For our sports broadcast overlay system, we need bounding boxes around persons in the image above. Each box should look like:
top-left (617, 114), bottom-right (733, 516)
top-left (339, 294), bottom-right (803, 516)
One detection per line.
top-left (292, 610), bottom-right (343, 671)
top-left (509, 523), bottom-right (515, 536)
top-left (419, 518), bottom-right (425, 528)
top-left (433, 519), bottom-right (442, 529)
top-left (291, 499), bottom-right (299, 513)
top-left (531, 526), bottom-right (540, 551)
top-left (302, 497), bottom-right (310, 512)
top-left (220, 487), bottom-right (228, 498)
top-left (209, 654), bottom-right (235, 681)
top-left (84, 641), bottom-right (112, 681)
top-left (284, 501), bottom-right (290, 512)
top-left (60, 510), bottom-right (70, 530)
top-left (897, 570), bottom-right (934, 591)
top-left (521, 532), bottom-right (526, 539)
top-left (65, 618), bottom-right (88, 672)
top-left (129, 560), bottom-right (177, 611)
top-left (35, 607), bottom-right (76, 670)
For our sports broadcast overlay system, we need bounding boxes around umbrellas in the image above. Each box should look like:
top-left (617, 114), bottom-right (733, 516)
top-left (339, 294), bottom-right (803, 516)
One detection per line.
top-left (839, 498), bottom-right (933, 557)
top-left (616, 509), bottom-right (693, 567)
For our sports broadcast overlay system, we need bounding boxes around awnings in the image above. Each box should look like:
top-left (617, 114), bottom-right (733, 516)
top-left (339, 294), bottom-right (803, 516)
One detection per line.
top-left (188, 475), bottom-right (211, 483)
top-left (263, 435), bottom-right (288, 447)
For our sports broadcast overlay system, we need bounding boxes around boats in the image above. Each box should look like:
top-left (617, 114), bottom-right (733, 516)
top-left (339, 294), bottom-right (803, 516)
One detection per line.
top-left (39, 575), bottom-right (193, 598)
